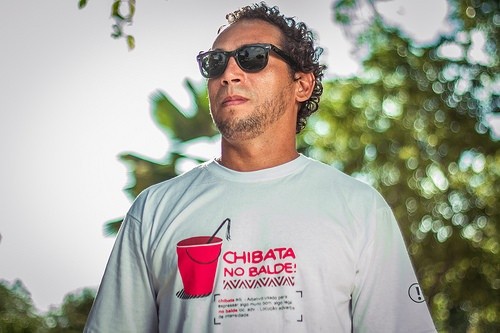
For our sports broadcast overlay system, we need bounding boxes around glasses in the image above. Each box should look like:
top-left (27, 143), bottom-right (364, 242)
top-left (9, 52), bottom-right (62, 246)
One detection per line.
top-left (197, 43), bottom-right (299, 79)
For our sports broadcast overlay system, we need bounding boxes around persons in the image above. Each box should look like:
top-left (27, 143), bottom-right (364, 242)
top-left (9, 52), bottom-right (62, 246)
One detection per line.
top-left (79, 0), bottom-right (441, 333)
